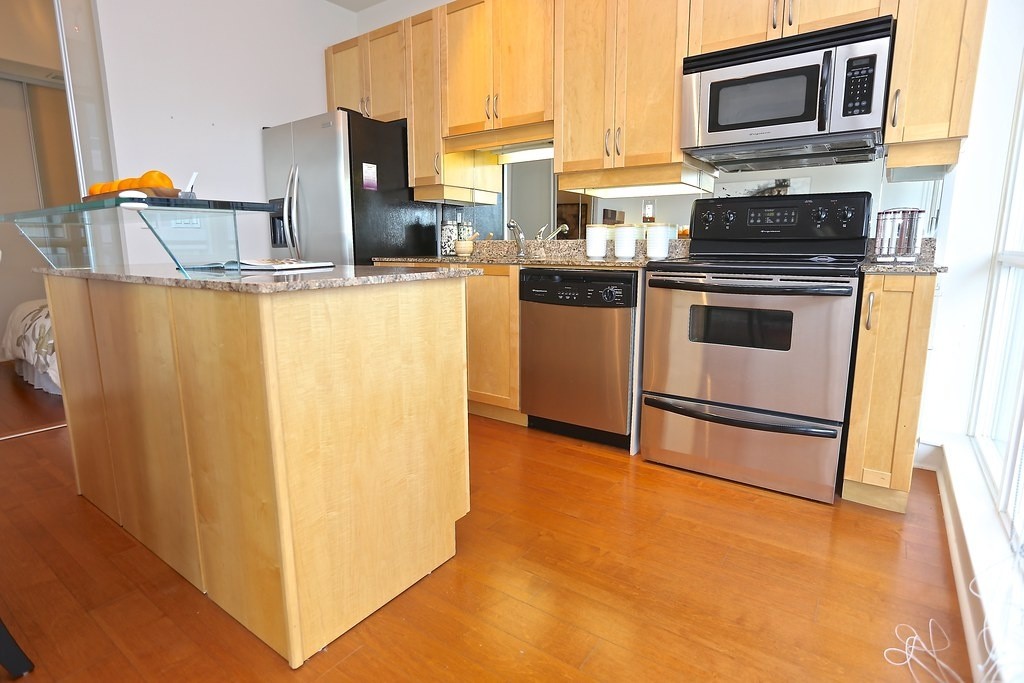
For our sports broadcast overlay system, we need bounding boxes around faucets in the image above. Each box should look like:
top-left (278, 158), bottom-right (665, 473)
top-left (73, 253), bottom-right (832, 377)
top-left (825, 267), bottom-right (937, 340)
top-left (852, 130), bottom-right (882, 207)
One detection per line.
top-left (507, 219), bottom-right (527, 259)
top-left (535, 223), bottom-right (569, 241)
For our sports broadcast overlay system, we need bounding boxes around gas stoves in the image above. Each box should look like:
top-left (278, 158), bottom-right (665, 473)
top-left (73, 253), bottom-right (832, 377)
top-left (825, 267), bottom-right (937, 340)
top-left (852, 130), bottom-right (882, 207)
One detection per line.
top-left (644, 191), bottom-right (872, 279)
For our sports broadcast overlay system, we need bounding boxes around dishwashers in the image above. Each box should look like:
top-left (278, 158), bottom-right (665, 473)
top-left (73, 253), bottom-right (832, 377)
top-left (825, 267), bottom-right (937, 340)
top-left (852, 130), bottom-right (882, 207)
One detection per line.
top-left (514, 268), bottom-right (640, 449)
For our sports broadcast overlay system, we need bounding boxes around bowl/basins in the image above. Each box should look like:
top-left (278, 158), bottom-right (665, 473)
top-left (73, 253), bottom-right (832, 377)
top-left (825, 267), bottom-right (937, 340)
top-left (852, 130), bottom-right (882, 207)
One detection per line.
top-left (456, 241), bottom-right (474, 257)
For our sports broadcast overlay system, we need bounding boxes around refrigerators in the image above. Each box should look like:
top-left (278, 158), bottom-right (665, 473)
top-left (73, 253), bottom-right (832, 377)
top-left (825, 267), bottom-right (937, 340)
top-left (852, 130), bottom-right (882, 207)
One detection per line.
top-left (261, 107), bottom-right (438, 266)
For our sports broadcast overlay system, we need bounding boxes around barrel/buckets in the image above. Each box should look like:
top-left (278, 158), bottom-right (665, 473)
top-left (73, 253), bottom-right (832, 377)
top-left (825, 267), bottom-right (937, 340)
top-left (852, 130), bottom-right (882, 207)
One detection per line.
top-left (873, 209), bottom-right (926, 256)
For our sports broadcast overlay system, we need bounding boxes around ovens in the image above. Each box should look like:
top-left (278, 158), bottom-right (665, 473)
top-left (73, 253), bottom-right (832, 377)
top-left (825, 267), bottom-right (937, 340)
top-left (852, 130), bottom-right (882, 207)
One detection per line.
top-left (637, 270), bottom-right (859, 507)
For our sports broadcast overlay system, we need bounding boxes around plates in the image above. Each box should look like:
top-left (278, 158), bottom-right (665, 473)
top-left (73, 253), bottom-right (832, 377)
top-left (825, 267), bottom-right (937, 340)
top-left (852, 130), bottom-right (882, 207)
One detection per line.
top-left (77, 187), bottom-right (181, 200)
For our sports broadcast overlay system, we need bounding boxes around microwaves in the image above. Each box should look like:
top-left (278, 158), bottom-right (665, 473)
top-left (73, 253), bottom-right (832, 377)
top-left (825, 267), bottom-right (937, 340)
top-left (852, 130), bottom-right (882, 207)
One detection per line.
top-left (678, 11), bottom-right (886, 173)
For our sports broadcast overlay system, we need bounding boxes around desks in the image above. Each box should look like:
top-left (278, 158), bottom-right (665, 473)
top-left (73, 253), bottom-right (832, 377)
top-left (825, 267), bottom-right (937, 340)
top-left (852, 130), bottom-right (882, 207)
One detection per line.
top-left (30, 254), bottom-right (476, 670)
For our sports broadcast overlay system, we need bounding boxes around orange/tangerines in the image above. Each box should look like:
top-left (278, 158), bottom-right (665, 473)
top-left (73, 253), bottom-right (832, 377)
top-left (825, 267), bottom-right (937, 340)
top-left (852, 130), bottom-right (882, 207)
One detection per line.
top-left (88, 171), bottom-right (174, 196)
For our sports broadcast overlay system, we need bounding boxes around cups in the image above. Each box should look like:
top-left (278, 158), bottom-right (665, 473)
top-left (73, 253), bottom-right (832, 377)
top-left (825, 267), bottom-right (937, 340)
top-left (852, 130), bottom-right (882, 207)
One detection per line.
top-left (182, 192), bottom-right (196, 199)
top-left (583, 223), bottom-right (670, 263)
top-left (642, 199), bottom-right (655, 222)
top-left (606, 222), bottom-right (688, 240)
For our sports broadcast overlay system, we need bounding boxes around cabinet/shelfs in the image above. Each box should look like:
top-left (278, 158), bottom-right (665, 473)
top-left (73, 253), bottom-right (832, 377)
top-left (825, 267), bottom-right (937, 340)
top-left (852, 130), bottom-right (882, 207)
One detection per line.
top-left (321, 0), bottom-right (994, 207)
